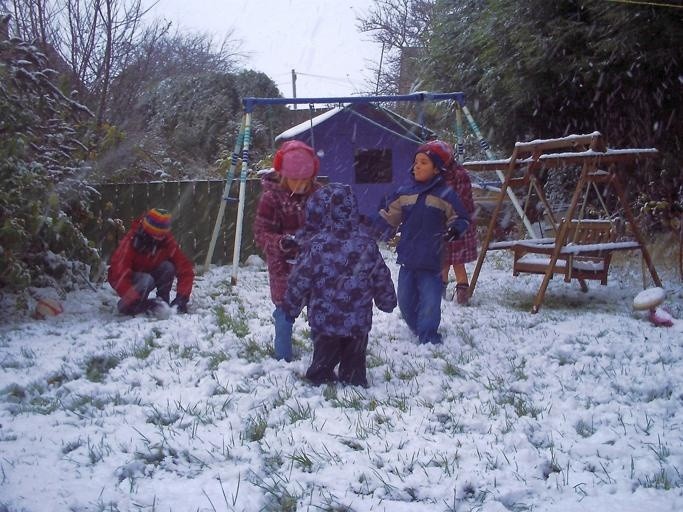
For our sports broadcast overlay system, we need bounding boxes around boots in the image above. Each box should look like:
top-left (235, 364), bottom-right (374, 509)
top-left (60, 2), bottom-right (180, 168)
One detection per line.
top-left (441, 282), bottom-right (469, 307)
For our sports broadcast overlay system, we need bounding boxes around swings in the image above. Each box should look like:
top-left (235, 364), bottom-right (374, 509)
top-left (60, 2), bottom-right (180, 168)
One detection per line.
top-left (267, 91), bottom-right (425, 250)
top-left (512, 146), bottom-right (620, 286)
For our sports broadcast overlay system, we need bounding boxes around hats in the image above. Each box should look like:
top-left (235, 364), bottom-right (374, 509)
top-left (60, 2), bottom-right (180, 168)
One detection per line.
top-left (279, 142), bottom-right (314, 179)
top-left (416, 140), bottom-right (454, 169)
top-left (142, 208), bottom-right (173, 238)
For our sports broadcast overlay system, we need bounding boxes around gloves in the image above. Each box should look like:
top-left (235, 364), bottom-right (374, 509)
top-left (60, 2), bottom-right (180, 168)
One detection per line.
top-left (169, 295), bottom-right (189, 313)
top-left (358, 215), bottom-right (371, 227)
top-left (286, 314), bottom-right (295, 324)
top-left (131, 299), bottom-right (157, 312)
top-left (281, 235), bottom-right (298, 249)
top-left (443, 227), bottom-right (458, 242)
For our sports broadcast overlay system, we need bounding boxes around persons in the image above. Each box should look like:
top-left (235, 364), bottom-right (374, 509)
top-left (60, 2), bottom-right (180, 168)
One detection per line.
top-left (106, 206), bottom-right (195, 317)
top-left (251, 139), bottom-right (327, 361)
top-left (365, 138), bottom-right (472, 346)
top-left (281, 181), bottom-right (397, 386)
top-left (438, 149), bottom-right (477, 305)
